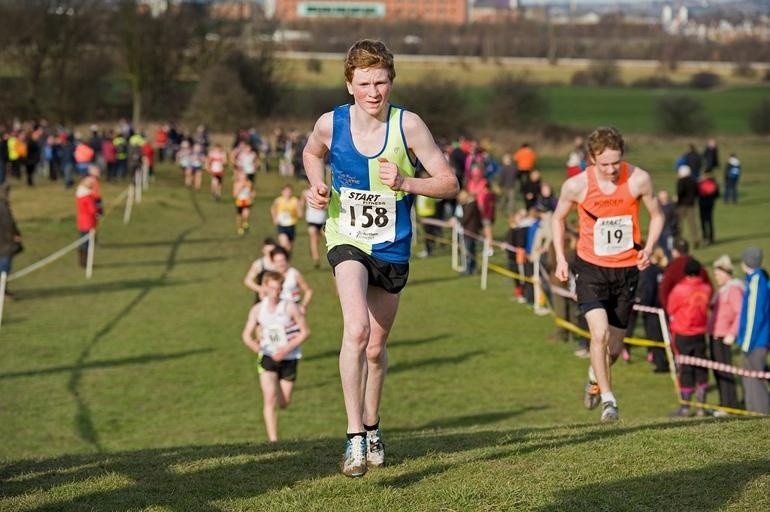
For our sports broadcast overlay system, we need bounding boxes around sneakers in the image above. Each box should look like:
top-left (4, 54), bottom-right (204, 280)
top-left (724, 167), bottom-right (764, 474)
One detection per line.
top-left (342, 436), bottom-right (367, 477)
top-left (367, 423), bottom-right (385, 467)
top-left (600, 401), bottom-right (618, 423)
top-left (585, 379), bottom-right (600, 409)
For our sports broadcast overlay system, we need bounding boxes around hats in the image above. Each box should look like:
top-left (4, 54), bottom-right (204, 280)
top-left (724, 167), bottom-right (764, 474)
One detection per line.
top-left (743, 249), bottom-right (763, 269)
top-left (712, 254), bottom-right (734, 273)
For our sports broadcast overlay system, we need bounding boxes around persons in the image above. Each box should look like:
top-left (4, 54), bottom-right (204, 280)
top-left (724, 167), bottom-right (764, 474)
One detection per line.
top-left (0, 175), bottom-right (24, 302)
top-left (262, 127), bottom-right (317, 180)
top-left (298, 175), bottom-right (329, 268)
top-left (241, 236), bottom-right (313, 444)
top-left (570, 238), bottom-right (769, 418)
top-left (551, 125), bottom-right (666, 422)
top-left (675, 139), bottom-right (740, 243)
top-left (74, 164), bottom-right (104, 267)
top-left (1, 116), bottom-right (155, 188)
top-left (301, 40), bottom-right (461, 477)
top-left (657, 190), bottom-right (680, 258)
top-left (408, 134), bottom-right (588, 314)
top-left (156, 123), bottom-right (229, 198)
top-left (230, 124), bottom-right (268, 238)
top-left (270, 183), bottom-right (304, 261)
